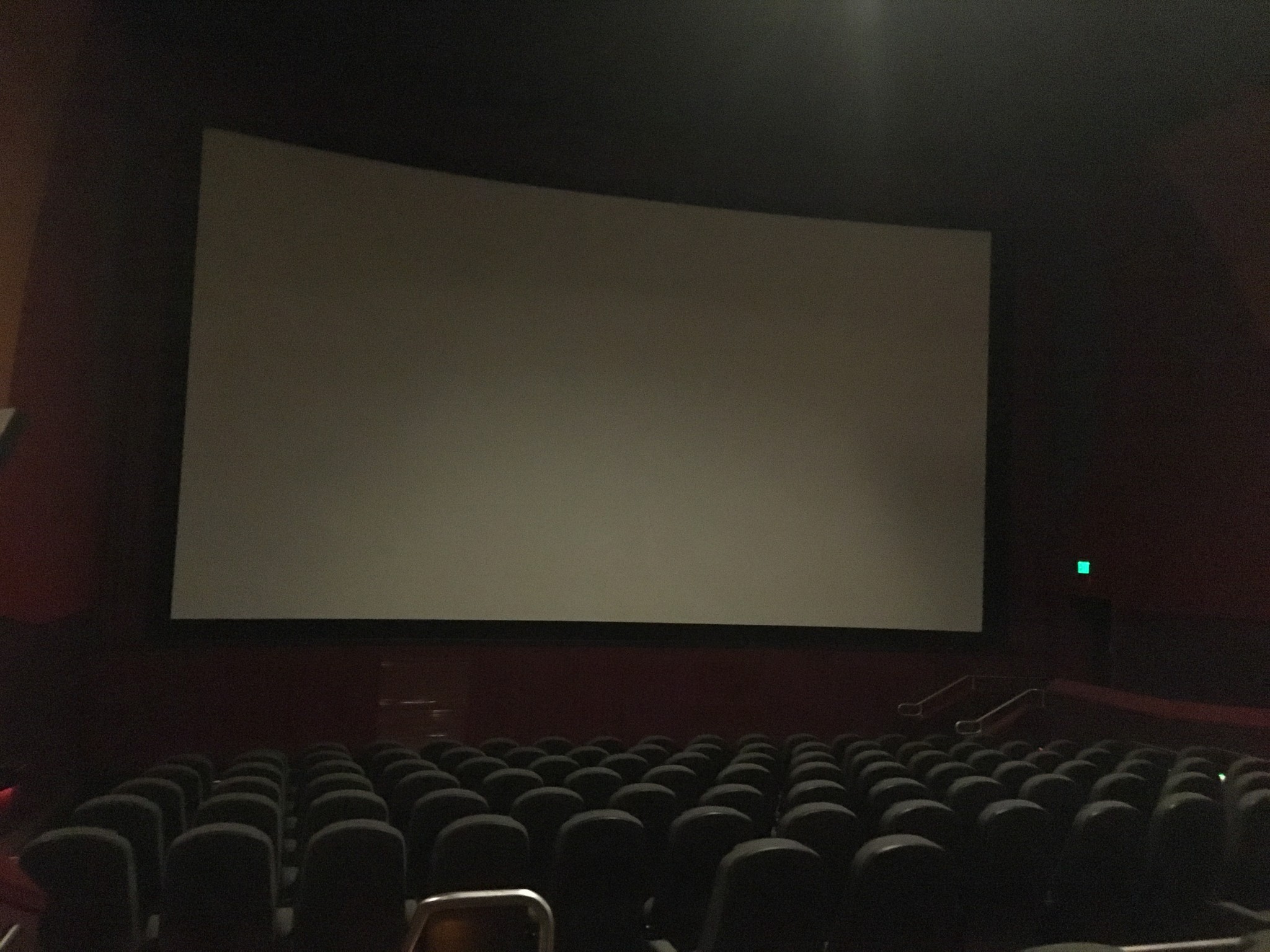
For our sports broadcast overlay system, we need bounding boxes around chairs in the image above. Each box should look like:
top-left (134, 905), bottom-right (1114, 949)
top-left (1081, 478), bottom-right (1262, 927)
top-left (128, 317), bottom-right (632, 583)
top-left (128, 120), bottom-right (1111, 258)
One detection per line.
top-left (5, 723), bottom-right (1270, 952)
